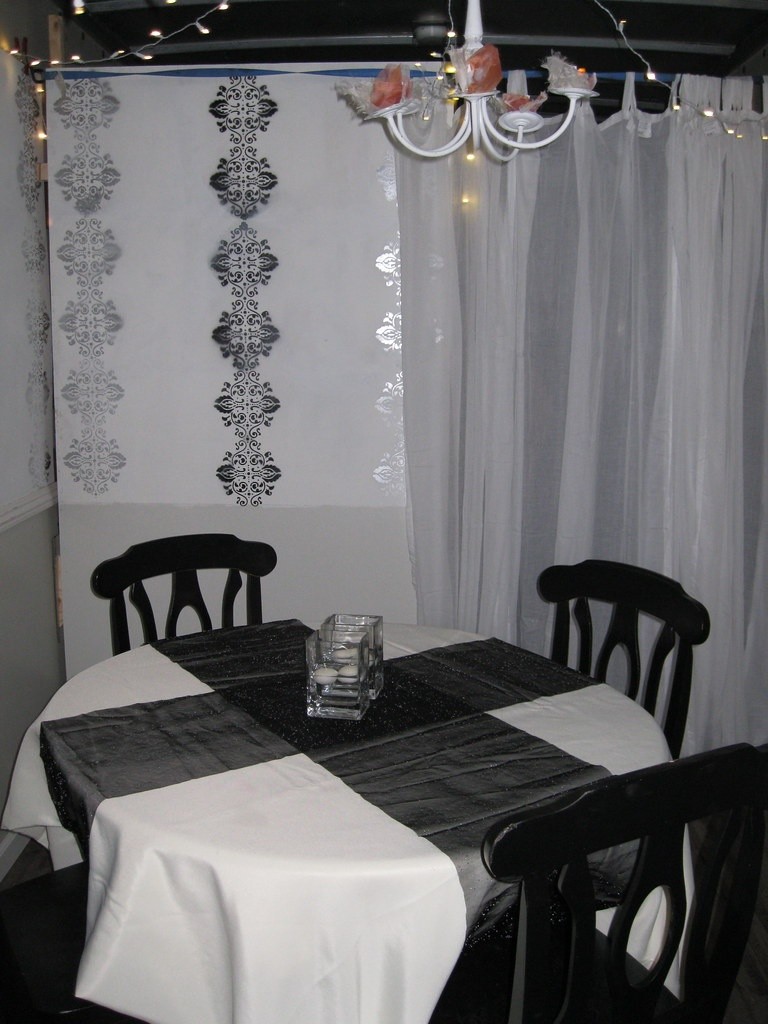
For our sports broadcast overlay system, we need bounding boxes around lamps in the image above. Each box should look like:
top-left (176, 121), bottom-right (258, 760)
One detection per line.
top-left (329, 1), bottom-right (603, 162)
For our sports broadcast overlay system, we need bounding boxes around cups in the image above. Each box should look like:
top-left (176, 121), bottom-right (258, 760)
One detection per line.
top-left (320, 613), bottom-right (384, 699)
top-left (304, 629), bottom-right (370, 719)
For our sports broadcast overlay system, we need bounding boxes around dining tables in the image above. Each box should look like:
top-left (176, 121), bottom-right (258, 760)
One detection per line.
top-left (1, 617), bottom-right (687, 1024)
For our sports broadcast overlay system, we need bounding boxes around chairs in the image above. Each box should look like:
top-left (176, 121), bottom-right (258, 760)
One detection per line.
top-left (89, 533), bottom-right (277, 654)
top-left (536, 558), bottom-right (713, 762)
top-left (1, 857), bottom-right (129, 1024)
top-left (431, 740), bottom-right (767, 1023)
